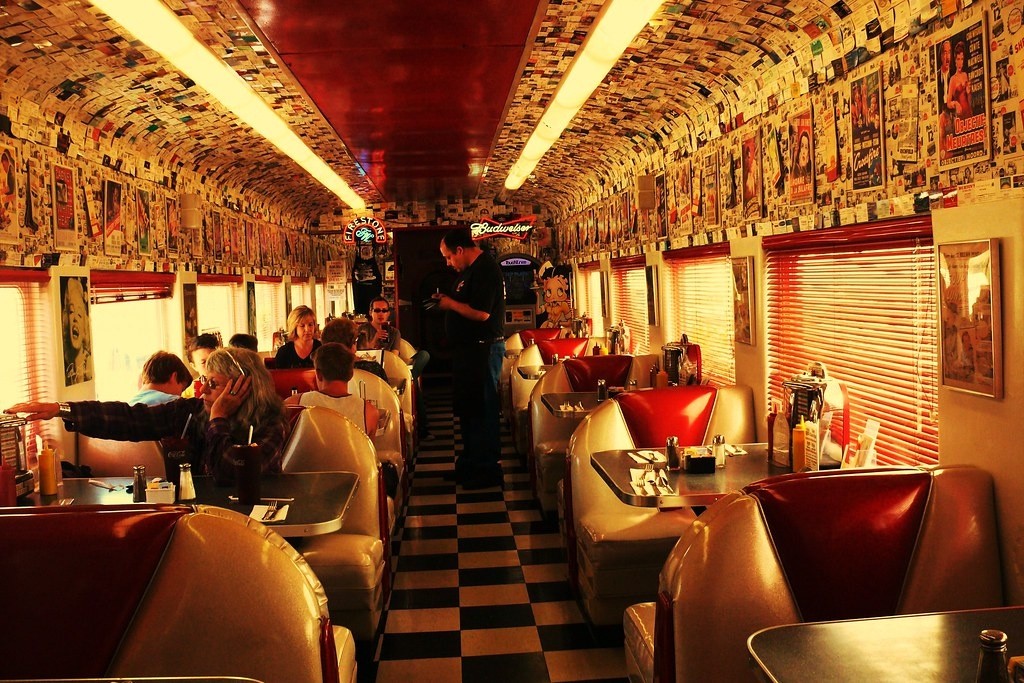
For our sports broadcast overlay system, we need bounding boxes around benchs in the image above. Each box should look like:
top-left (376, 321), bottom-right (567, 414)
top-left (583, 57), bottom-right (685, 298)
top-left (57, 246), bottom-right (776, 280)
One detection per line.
top-left (501, 328), bottom-right (1004, 683)
top-left (0, 337), bottom-right (421, 683)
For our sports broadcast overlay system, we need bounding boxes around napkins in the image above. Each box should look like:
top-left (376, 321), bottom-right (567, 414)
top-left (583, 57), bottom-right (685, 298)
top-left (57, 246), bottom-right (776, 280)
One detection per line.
top-left (629, 481), bottom-right (676, 497)
top-left (559, 403), bottom-right (584, 412)
top-left (248, 505), bottom-right (289, 523)
top-left (707, 445), bottom-right (749, 455)
top-left (629, 468), bottom-right (667, 482)
top-left (626, 450), bottom-right (667, 464)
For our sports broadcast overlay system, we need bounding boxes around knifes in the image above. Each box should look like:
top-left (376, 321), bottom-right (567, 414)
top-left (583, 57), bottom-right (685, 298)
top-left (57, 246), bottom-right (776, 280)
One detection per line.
top-left (648, 480), bottom-right (661, 496)
top-left (631, 450), bottom-right (656, 465)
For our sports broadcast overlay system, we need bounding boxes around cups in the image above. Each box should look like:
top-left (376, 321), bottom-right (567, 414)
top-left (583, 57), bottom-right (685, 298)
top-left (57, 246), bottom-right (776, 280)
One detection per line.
top-left (359, 314), bottom-right (366, 319)
top-left (381, 321), bottom-right (390, 343)
top-left (194, 380), bottom-right (205, 398)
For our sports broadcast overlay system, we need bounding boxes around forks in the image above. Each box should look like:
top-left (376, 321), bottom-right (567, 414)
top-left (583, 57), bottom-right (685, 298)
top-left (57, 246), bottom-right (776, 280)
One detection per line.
top-left (637, 481), bottom-right (648, 495)
top-left (564, 401), bottom-right (569, 410)
top-left (640, 464), bottom-right (654, 479)
top-left (732, 445), bottom-right (741, 453)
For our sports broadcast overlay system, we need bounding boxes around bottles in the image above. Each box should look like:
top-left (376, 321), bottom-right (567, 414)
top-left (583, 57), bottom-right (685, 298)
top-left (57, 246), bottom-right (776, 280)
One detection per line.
top-left (650, 365), bottom-right (668, 388)
top-left (0, 455), bottom-right (17, 507)
top-left (552, 354), bottom-right (570, 365)
top-left (666, 436), bottom-right (681, 471)
top-left (713, 435), bottom-right (725, 468)
top-left (792, 415), bottom-right (806, 472)
top-left (39, 442), bottom-right (58, 495)
top-left (598, 379), bottom-right (607, 401)
top-left (290, 385), bottom-right (298, 396)
top-left (180, 463), bottom-right (196, 500)
top-left (976, 630), bottom-right (1009, 683)
top-left (629, 379), bottom-right (637, 391)
top-left (768, 404), bottom-right (778, 461)
top-left (529, 339), bottom-right (534, 346)
top-left (593, 343), bottom-right (608, 356)
top-left (565, 330), bottom-right (576, 338)
top-left (573, 354), bottom-right (577, 359)
top-left (133, 466), bottom-right (146, 502)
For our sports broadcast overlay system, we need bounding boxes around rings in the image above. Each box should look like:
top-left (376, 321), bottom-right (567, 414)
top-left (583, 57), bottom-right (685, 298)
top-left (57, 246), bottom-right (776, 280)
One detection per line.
top-left (230, 391), bottom-right (235, 395)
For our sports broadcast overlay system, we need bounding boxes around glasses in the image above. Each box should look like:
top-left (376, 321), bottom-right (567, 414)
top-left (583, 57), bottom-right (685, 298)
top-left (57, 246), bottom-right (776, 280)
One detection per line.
top-left (370, 308), bottom-right (389, 313)
top-left (199, 375), bottom-right (227, 389)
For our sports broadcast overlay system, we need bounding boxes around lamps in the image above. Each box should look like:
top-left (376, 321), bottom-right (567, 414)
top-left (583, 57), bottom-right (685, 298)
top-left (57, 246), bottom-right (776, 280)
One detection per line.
top-left (498, 0), bottom-right (666, 203)
top-left (88, 0), bottom-right (365, 209)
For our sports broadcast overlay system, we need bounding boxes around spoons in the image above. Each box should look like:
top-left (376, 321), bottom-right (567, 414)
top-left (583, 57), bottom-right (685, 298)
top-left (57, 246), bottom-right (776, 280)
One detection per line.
top-left (660, 477), bottom-right (675, 493)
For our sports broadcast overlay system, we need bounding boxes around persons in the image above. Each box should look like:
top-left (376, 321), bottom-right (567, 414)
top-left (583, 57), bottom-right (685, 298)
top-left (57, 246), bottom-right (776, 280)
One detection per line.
top-left (358, 297), bottom-right (435, 440)
top-left (789, 125), bottom-right (811, 185)
top-left (321, 317), bottom-right (389, 384)
top-left (3, 348), bottom-right (290, 486)
top-left (274, 305), bottom-right (322, 368)
top-left (181, 333), bottom-right (219, 398)
top-left (127, 351), bottom-right (193, 406)
top-left (285, 342), bottom-right (379, 445)
top-left (432, 229), bottom-right (506, 490)
top-left (0, 153), bottom-right (16, 202)
top-left (851, 41), bottom-right (1024, 190)
top-left (230, 334), bottom-right (258, 353)
top-left (62, 278), bottom-right (92, 385)
top-left (943, 303), bottom-right (975, 382)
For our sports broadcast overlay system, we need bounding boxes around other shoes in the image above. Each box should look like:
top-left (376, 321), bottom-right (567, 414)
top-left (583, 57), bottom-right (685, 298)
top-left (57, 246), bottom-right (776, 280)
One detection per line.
top-left (421, 434), bottom-right (436, 441)
top-left (456, 469), bottom-right (505, 490)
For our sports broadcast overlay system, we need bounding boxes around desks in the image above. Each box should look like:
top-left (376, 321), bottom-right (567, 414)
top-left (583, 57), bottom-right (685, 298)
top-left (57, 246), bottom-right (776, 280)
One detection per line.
top-left (747, 604), bottom-right (1024, 683)
top-left (375, 407), bottom-right (390, 438)
top-left (541, 391), bottom-right (598, 418)
top-left (517, 366), bottom-right (550, 380)
top-left (504, 348), bottom-right (525, 360)
top-left (589, 443), bottom-right (792, 517)
top-left (18, 472), bottom-right (360, 537)
top-left (388, 377), bottom-right (407, 395)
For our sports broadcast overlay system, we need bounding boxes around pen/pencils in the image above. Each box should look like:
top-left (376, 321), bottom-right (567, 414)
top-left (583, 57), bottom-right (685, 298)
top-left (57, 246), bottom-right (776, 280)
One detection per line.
top-left (437, 288), bottom-right (439, 293)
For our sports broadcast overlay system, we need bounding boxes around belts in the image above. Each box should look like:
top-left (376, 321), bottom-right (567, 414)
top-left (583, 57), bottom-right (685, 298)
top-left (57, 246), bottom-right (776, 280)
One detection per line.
top-left (475, 335), bottom-right (504, 348)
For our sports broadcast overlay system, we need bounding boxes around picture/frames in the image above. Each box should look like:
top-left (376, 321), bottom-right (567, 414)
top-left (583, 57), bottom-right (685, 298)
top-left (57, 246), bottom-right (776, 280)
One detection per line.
top-left (645, 264), bottom-right (660, 327)
top-left (599, 270), bottom-right (608, 318)
top-left (732, 256), bottom-right (756, 346)
top-left (569, 272), bottom-right (577, 310)
top-left (937, 238), bottom-right (1004, 400)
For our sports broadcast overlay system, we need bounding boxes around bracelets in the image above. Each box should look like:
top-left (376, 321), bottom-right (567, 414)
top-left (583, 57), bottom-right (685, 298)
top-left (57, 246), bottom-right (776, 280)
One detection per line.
top-left (56, 402), bottom-right (71, 417)
